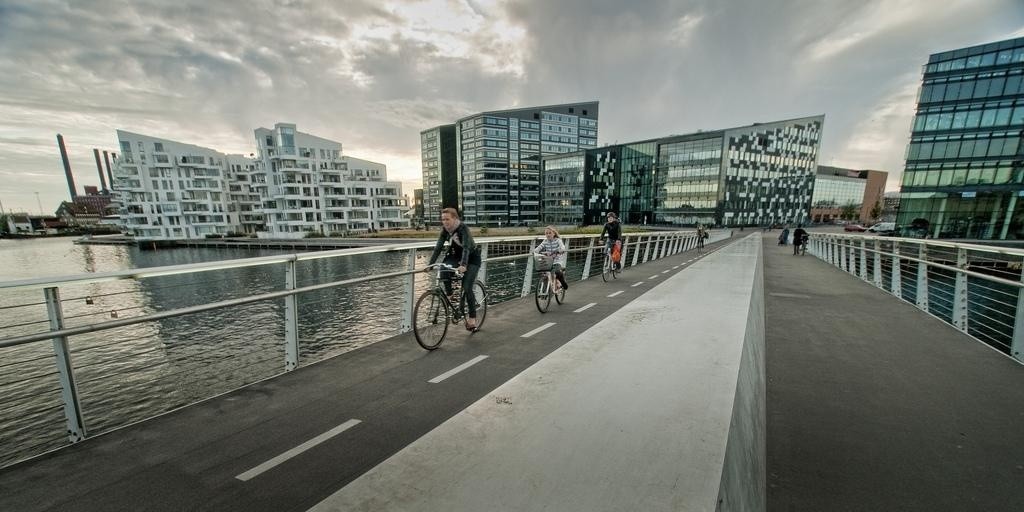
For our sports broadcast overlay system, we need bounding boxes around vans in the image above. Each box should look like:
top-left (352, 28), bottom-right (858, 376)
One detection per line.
top-left (864, 222), bottom-right (895, 234)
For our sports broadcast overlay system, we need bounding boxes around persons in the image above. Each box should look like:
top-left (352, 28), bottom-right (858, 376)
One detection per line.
top-left (696, 222), bottom-right (705, 248)
top-left (532, 225), bottom-right (568, 291)
top-left (792, 224), bottom-right (811, 255)
top-left (782, 226), bottom-right (789, 246)
top-left (425, 208), bottom-right (481, 330)
top-left (598, 212), bottom-right (622, 273)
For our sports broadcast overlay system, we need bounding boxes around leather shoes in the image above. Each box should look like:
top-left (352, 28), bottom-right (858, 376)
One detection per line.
top-left (466, 317), bottom-right (476, 331)
top-left (441, 295), bottom-right (454, 307)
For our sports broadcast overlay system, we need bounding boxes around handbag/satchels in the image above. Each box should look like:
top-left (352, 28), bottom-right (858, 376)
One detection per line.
top-left (611, 245), bottom-right (621, 262)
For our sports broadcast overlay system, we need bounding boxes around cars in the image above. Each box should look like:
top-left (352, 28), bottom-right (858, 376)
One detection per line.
top-left (844, 225), bottom-right (867, 232)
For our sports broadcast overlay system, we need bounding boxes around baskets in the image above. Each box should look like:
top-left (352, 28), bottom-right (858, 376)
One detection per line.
top-left (534, 255), bottom-right (554, 271)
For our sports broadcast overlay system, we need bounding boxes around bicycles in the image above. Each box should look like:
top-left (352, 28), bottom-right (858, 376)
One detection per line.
top-left (528, 249), bottom-right (566, 314)
top-left (798, 234), bottom-right (810, 257)
top-left (696, 233), bottom-right (705, 253)
top-left (596, 241), bottom-right (621, 284)
top-left (412, 260), bottom-right (487, 351)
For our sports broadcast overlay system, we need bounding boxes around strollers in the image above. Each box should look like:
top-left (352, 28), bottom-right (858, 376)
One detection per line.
top-left (776, 233), bottom-right (786, 246)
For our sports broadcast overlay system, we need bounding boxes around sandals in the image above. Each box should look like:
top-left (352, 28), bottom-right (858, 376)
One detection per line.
top-left (557, 284), bottom-right (563, 291)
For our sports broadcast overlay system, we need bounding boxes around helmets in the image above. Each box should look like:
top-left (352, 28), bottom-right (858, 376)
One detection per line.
top-left (607, 211), bottom-right (616, 218)
